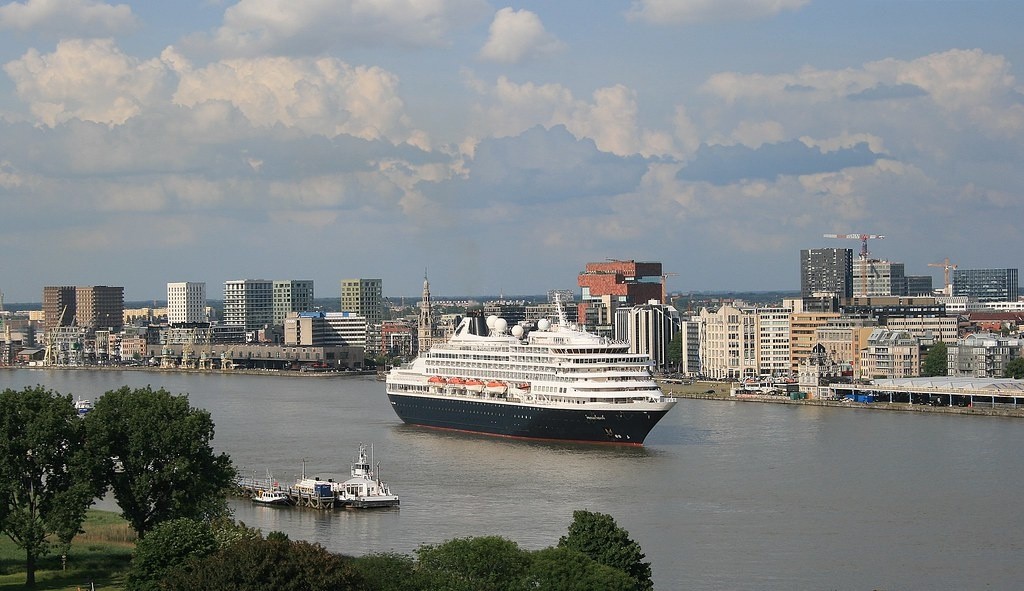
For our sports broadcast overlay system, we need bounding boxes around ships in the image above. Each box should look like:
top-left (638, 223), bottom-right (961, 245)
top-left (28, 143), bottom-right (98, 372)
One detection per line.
top-left (385, 290), bottom-right (679, 448)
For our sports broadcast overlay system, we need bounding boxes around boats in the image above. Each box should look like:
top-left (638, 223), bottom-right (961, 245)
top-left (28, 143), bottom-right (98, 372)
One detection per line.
top-left (428, 375), bottom-right (447, 389)
top-left (336, 440), bottom-right (401, 509)
top-left (251, 457), bottom-right (356, 509)
top-left (465, 377), bottom-right (485, 392)
top-left (512, 383), bottom-right (531, 397)
top-left (445, 376), bottom-right (467, 390)
top-left (76, 395), bottom-right (94, 413)
top-left (486, 380), bottom-right (507, 395)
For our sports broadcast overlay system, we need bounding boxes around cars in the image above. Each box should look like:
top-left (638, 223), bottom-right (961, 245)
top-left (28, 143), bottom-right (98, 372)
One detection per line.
top-left (659, 368), bottom-right (798, 398)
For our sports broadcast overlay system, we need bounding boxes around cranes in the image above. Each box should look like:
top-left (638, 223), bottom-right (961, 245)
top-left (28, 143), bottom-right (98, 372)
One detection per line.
top-left (220, 344), bottom-right (238, 371)
top-left (43, 304), bottom-right (68, 367)
top-left (198, 324), bottom-right (213, 369)
top-left (927, 258), bottom-right (959, 297)
top-left (180, 327), bottom-right (198, 369)
top-left (161, 324), bottom-right (177, 369)
top-left (822, 233), bottom-right (885, 297)
